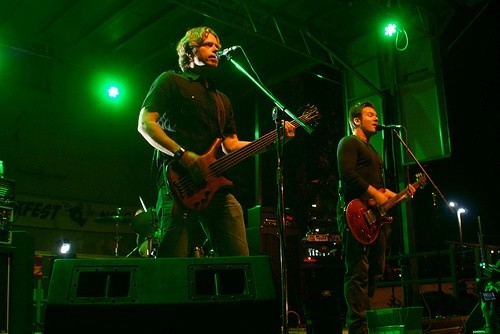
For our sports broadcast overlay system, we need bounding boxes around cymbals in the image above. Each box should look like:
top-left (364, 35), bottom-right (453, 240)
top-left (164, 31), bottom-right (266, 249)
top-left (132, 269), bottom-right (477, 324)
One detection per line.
top-left (94, 216), bottom-right (131, 223)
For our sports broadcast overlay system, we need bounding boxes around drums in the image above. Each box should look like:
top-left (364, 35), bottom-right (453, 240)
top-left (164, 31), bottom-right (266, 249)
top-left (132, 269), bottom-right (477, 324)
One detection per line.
top-left (190, 243), bottom-right (206, 257)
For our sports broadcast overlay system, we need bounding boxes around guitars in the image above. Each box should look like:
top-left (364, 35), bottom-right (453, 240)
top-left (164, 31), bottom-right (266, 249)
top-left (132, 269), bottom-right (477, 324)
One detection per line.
top-left (345, 172), bottom-right (430, 246)
top-left (164, 103), bottom-right (321, 212)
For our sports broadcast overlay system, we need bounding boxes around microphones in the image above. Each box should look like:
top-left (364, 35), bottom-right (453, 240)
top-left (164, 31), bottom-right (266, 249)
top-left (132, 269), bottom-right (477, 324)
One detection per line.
top-left (216, 46), bottom-right (240, 60)
top-left (376, 124), bottom-right (402, 131)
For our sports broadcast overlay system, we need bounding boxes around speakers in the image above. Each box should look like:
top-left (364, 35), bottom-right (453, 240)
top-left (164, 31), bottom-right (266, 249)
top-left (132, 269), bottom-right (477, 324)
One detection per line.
top-left (41, 256), bottom-right (282, 334)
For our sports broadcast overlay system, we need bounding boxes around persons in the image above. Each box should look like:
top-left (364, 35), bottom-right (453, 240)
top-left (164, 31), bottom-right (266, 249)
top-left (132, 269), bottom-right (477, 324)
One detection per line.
top-left (138, 26), bottom-right (250, 258)
top-left (337, 102), bottom-right (416, 334)
